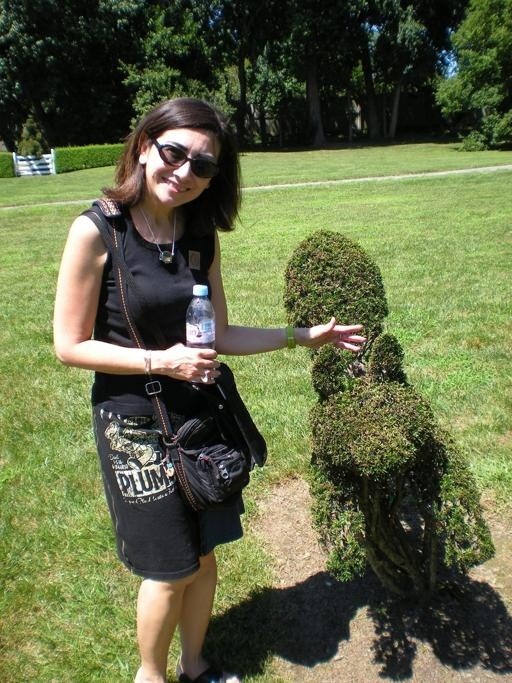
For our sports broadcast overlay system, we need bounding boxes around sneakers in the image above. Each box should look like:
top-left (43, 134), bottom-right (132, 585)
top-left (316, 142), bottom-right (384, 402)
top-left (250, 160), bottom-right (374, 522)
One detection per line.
top-left (174, 652), bottom-right (241, 683)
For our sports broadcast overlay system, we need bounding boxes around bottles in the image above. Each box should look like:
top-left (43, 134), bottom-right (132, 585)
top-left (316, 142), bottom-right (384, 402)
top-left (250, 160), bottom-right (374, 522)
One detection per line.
top-left (184, 284), bottom-right (218, 389)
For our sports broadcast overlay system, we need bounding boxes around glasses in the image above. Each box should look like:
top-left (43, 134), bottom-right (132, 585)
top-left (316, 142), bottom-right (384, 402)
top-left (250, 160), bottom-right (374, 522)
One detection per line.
top-left (145, 129), bottom-right (223, 180)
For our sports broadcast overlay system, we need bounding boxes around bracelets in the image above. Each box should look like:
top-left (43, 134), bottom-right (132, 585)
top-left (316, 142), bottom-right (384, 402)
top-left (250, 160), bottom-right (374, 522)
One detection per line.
top-left (285, 323), bottom-right (297, 350)
top-left (142, 347), bottom-right (153, 375)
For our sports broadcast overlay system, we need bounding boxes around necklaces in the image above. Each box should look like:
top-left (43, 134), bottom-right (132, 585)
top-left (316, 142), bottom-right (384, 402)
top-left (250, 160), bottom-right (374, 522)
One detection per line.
top-left (135, 198), bottom-right (182, 266)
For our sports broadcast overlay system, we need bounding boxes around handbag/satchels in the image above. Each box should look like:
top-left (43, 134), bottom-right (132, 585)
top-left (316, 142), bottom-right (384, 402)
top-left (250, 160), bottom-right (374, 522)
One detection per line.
top-left (159, 400), bottom-right (251, 514)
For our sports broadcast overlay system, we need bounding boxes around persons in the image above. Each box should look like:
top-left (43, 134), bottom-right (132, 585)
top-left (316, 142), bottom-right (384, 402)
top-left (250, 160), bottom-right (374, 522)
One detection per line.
top-left (47, 93), bottom-right (368, 683)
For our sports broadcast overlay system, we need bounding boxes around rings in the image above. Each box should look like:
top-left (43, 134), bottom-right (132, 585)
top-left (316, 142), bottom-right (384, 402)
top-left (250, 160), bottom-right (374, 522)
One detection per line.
top-left (200, 367), bottom-right (211, 383)
